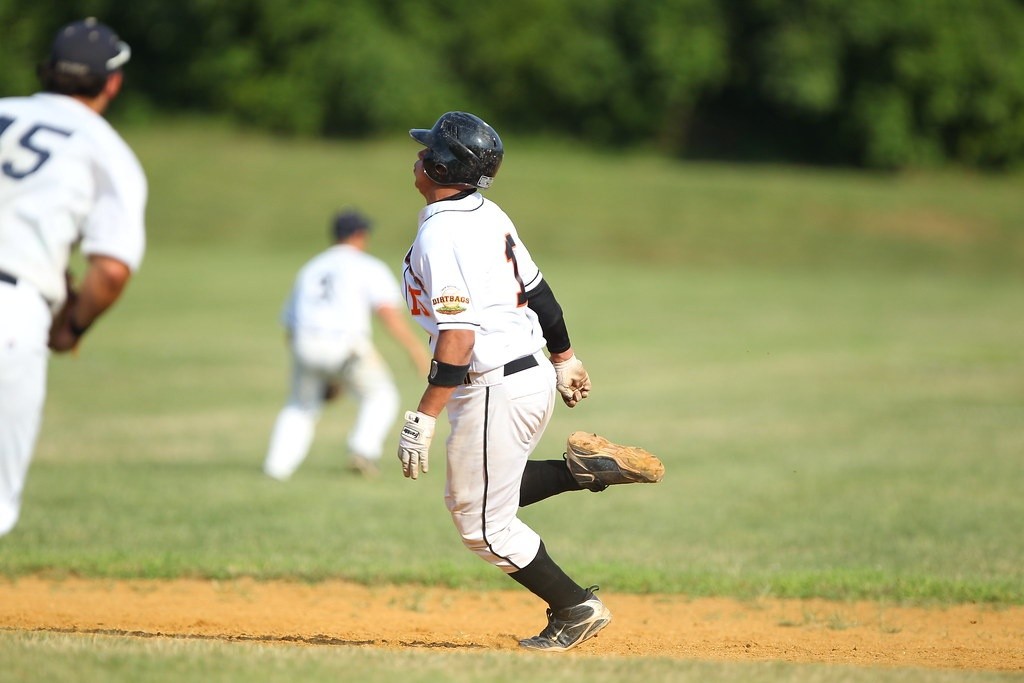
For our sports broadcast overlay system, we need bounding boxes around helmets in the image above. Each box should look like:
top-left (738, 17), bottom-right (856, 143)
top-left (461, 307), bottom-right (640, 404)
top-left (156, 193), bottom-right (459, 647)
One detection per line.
top-left (408, 109), bottom-right (504, 191)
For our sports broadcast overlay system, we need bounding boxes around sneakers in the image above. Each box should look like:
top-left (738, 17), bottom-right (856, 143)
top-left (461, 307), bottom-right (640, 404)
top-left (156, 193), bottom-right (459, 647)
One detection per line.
top-left (519, 591), bottom-right (613, 654)
top-left (566, 430), bottom-right (665, 494)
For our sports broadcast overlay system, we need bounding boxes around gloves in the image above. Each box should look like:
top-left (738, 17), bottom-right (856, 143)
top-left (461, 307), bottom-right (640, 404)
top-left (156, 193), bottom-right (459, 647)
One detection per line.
top-left (553, 352), bottom-right (592, 409)
top-left (397, 409), bottom-right (436, 480)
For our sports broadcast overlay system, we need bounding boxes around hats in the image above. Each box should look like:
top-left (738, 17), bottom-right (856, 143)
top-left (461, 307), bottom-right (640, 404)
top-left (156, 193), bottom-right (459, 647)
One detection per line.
top-left (40, 16), bottom-right (132, 74)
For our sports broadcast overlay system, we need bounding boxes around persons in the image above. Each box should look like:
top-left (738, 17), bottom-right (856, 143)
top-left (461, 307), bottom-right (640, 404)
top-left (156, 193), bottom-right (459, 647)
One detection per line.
top-left (0, 15), bottom-right (153, 541)
top-left (264, 204), bottom-right (435, 485)
top-left (397, 109), bottom-right (668, 648)
